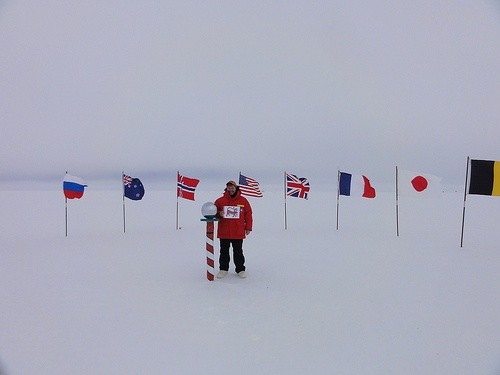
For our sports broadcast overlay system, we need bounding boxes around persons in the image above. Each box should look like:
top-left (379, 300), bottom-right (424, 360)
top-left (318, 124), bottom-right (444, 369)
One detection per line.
top-left (213, 181), bottom-right (253, 279)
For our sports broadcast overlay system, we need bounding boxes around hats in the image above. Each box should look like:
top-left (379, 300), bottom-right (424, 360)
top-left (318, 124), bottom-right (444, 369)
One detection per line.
top-left (226, 181), bottom-right (236, 186)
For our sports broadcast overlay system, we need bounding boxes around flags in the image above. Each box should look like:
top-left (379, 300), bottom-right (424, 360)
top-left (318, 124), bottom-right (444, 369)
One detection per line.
top-left (468, 159), bottom-right (500, 196)
top-left (177, 174), bottom-right (200, 202)
top-left (239, 174), bottom-right (263, 198)
top-left (122, 174), bottom-right (145, 201)
top-left (397, 167), bottom-right (442, 197)
top-left (63, 174), bottom-right (87, 200)
top-left (286, 173), bottom-right (310, 200)
top-left (338, 171), bottom-right (375, 200)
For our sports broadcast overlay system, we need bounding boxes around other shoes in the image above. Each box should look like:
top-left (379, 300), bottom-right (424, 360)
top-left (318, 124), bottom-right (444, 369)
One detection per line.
top-left (217, 270), bottom-right (228, 278)
top-left (238, 271), bottom-right (247, 278)
